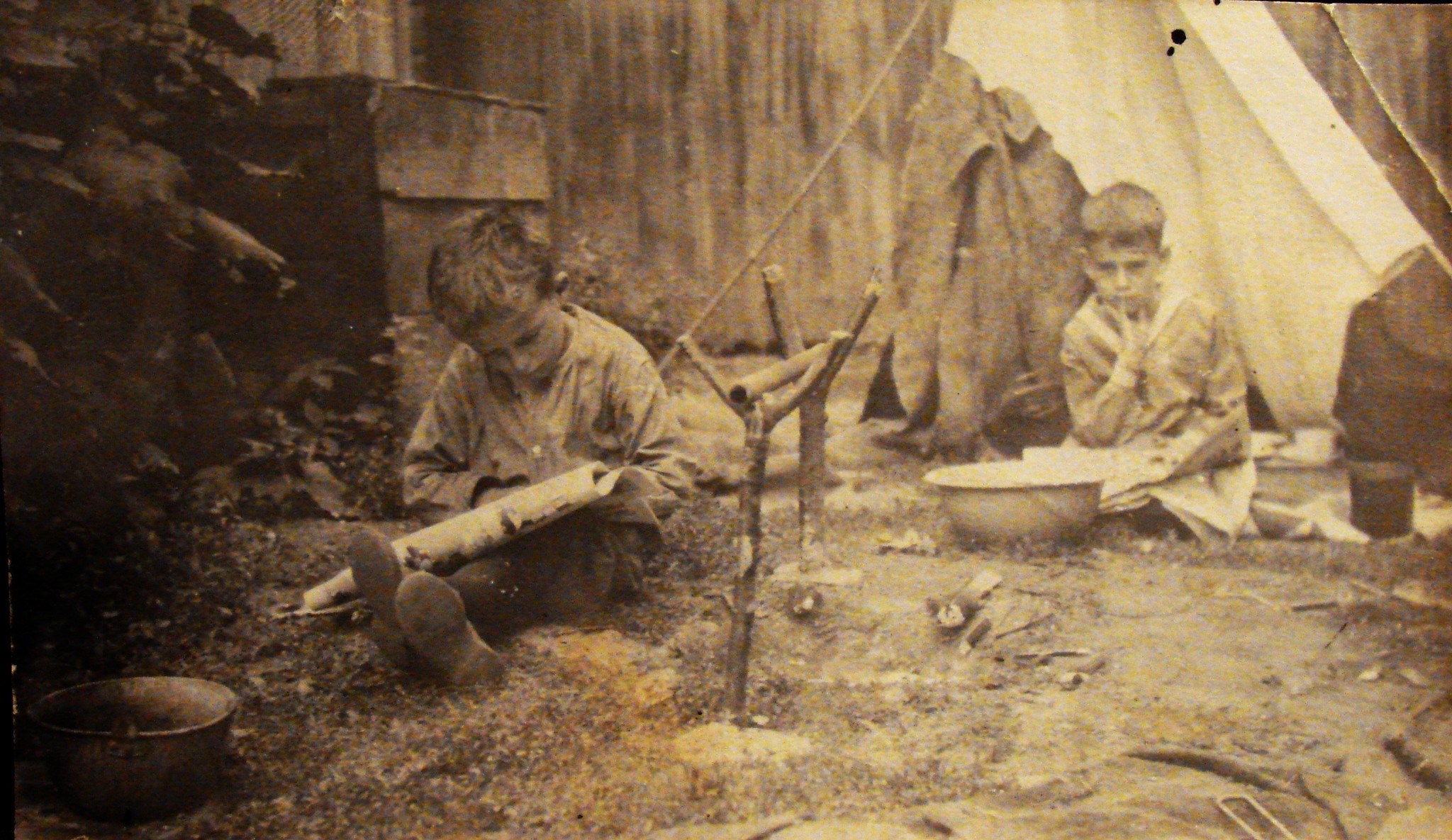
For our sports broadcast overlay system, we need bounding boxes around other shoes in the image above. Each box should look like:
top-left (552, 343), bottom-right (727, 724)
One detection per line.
top-left (395, 571), bottom-right (505, 690)
top-left (350, 525), bottom-right (406, 628)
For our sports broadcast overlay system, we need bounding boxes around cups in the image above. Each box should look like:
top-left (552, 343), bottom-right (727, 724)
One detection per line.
top-left (1349, 465), bottom-right (1417, 539)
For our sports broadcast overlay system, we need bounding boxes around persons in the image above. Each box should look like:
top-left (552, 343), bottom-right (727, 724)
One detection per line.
top-left (1057, 182), bottom-right (1248, 467)
top-left (349, 203), bottom-right (701, 701)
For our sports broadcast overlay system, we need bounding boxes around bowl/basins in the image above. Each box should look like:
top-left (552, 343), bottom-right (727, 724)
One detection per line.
top-left (922, 457), bottom-right (1108, 558)
top-left (23, 674), bottom-right (237, 822)
